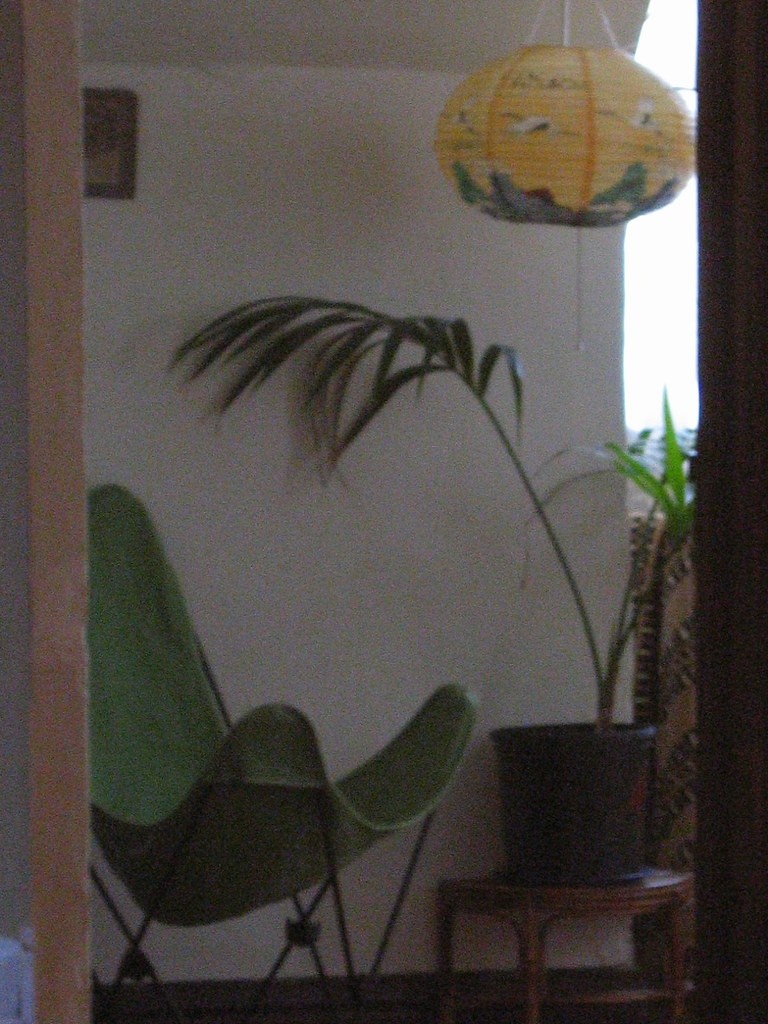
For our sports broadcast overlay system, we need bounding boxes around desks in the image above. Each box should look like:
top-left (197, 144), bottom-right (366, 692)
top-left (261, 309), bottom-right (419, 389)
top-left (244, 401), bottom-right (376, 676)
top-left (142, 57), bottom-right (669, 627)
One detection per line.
top-left (436, 868), bottom-right (697, 1024)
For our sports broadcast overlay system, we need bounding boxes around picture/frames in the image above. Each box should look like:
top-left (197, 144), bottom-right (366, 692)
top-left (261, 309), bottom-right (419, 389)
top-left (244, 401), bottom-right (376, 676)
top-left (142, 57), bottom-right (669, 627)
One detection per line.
top-left (80, 87), bottom-right (138, 201)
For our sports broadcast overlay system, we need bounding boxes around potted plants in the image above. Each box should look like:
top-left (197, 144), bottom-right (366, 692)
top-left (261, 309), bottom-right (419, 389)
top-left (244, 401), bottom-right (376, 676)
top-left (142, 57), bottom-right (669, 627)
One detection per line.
top-left (167, 293), bottom-right (700, 885)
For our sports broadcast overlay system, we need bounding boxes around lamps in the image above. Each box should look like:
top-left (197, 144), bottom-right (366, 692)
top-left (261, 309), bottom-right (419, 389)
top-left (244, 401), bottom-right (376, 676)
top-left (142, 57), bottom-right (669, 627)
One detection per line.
top-left (435, 1), bottom-right (691, 229)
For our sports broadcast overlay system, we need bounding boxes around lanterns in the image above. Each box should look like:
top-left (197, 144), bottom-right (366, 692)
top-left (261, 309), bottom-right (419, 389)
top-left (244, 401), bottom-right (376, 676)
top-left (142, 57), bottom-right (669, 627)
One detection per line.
top-left (433, 0), bottom-right (695, 229)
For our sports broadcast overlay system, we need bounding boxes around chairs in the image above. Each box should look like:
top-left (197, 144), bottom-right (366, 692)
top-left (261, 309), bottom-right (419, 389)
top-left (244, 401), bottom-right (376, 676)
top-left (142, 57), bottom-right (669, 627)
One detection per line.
top-left (80, 483), bottom-right (475, 1024)
